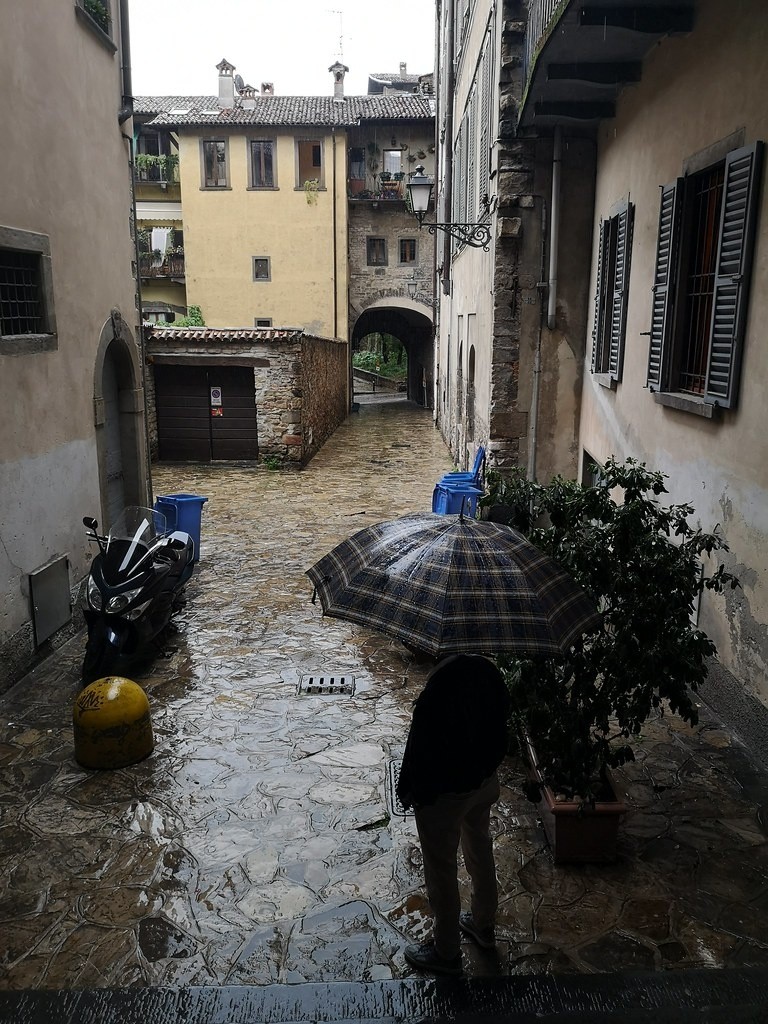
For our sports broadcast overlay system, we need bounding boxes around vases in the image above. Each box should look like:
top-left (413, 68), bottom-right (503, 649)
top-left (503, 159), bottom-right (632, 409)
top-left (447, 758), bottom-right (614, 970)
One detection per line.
top-left (350, 178), bottom-right (365, 194)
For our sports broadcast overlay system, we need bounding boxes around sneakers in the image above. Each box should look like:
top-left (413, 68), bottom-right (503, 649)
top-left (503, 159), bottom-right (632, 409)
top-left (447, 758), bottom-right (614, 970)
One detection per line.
top-left (406, 938), bottom-right (463, 973)
top-left (458, 910), bottom-right (497, 948)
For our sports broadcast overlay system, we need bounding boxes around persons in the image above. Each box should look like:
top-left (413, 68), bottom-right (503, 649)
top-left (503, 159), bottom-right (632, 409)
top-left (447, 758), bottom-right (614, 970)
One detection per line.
top-left (390, 655), bottom-right (526, 974)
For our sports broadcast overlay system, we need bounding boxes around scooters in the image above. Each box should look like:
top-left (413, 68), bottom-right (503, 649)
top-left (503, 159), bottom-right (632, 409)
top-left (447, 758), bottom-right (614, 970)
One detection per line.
top-left (80, 506), bottom-right (196, 689)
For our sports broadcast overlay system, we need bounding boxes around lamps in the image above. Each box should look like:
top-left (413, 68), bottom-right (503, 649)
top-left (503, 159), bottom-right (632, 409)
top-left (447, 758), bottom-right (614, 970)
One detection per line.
top-left (406, 165), bottom-right (492, 253)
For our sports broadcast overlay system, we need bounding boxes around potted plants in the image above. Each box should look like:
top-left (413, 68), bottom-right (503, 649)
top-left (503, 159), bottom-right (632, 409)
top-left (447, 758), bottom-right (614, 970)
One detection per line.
top-left (406, 154), bottom-right (416, 162)
top-left (84, 0), bottom-right (110, 34)
top-left (378, 172), bottom-right (391, 180)
top-left (478, 454), bottom-right (743, 867)
top-left (134, 153), bottom-right (178, 181)
top-left (304, 178), bottom-right (319, 206)
top-left (417, 149), bottom-right (426, 160)
top-left (426, 144), bottom-right (434, 154)
top-left (394, 172), bottom-right (405, 181)
top-left (135, 225), bottom-right (184, 277)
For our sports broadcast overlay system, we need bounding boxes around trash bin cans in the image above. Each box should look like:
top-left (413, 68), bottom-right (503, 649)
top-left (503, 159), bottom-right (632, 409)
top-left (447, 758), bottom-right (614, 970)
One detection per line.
top-left (432, 446), bottom-right (485, 518)
top-left (154, 494), bottom-right (208, 562)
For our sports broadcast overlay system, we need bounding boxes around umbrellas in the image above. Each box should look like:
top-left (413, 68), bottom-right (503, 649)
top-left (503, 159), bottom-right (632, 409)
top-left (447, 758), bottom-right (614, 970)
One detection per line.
top-left (300, 493), bottom-right (600, 658)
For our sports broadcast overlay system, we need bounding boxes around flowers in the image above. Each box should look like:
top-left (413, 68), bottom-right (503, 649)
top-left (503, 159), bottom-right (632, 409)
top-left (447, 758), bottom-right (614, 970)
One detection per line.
top-left (358, 188), bottom-right (398, 200)
